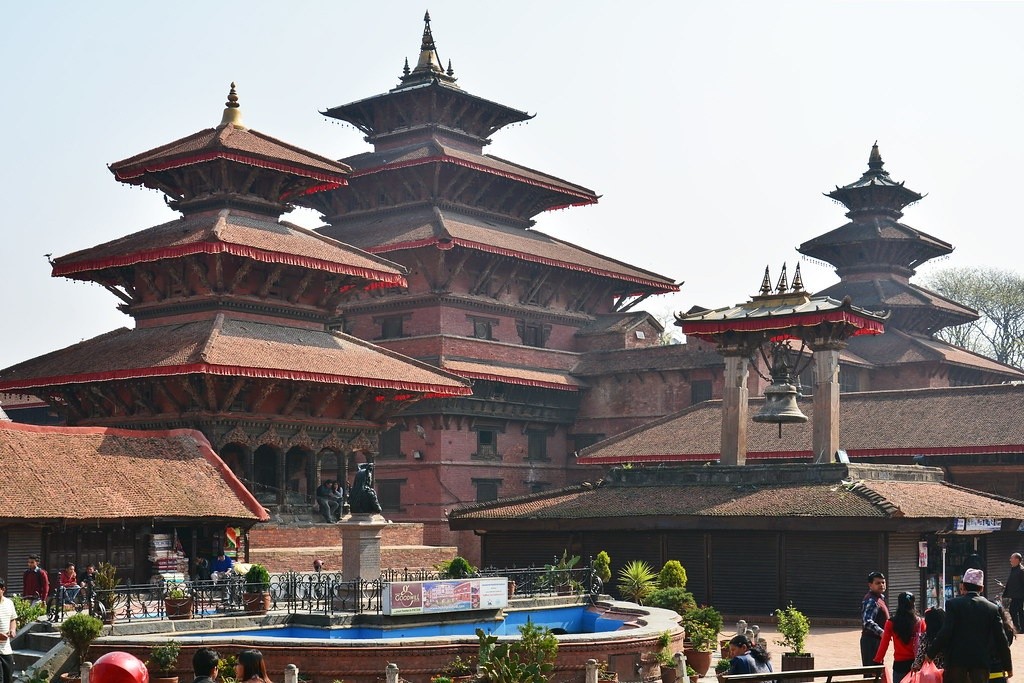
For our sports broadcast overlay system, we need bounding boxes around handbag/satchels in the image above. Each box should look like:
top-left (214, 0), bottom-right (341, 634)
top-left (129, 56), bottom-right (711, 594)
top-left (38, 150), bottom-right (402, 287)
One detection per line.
top-left (916, 659), bottom-right (944, 683)
top-left (900, 669), bottom-right (916, 683)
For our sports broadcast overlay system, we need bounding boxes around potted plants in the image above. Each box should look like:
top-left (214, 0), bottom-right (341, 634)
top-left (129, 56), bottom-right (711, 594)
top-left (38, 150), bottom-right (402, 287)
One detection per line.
top-left (58, 612), bottom-right (103, 683)
top-left (243, 563), bottom-right (270, 615)
top-left (163, 584), bottom-right (193, 620)
top-left (93, 560), bottom-right (122, 625)
top-left (214, 656), bottom-right (241, 683)
top-left (150, 637), bottom-right (182, 683)
top-left (445, 549), bottom-right (814, 683)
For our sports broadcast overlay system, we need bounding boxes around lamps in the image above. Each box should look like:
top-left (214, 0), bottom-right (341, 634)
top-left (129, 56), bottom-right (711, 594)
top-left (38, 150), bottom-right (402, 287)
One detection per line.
top-left (413, 450), bottom-right (421, 459)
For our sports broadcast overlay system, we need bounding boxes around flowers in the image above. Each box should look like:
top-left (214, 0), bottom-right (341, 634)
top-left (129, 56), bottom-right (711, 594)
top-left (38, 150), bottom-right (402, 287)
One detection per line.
top-left (431, 674), bottom-right (454, 683)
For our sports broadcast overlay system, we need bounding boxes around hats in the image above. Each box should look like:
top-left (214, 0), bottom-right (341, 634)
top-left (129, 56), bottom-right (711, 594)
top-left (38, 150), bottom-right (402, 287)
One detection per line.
top-left (963, 568), bottom-right (984, 587)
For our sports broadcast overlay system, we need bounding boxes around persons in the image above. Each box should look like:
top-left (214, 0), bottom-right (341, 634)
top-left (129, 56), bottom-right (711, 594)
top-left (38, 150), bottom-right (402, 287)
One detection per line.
top-left (1002, 553), bottom-right (1024, 633)
top-left (192, 648), bottom-right (218, 683)
top-left (926, 568), bottom-right (1013, 683)
top-left (53, 563), bottom-right (80, 607)
top-left (196, 555), bottom-right (210, 584)
top-left (728, 635), bottom-right (774, 683)
top-left (860, 572), bottom-right (892, 678)
top-left (989, 599), bottom-right (1016, 683)
top-left (873, 592), bottom-right (926, 683)
top-left (317, 479), bottom-right (351, 523)
top-left (911, 607), bottom-right (943, 677)
top-left (234, 649), bottom-right (272, 683)
top-left (0, 579), bottom-right (18, 683)
top-left (23, 554), bottom-right (49, 606)
top-left (349, 463), bottom-right (382, 513)
top-left (80, 564), bottom-right (100, 603)
top-left (209, 550), bottom-right (233, 594)
